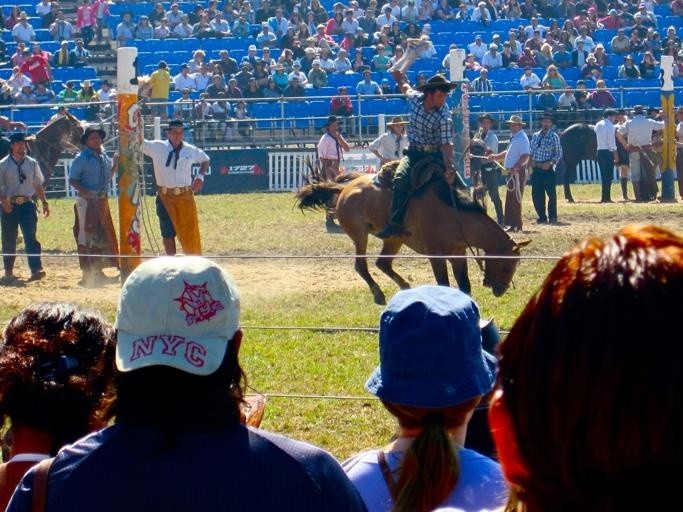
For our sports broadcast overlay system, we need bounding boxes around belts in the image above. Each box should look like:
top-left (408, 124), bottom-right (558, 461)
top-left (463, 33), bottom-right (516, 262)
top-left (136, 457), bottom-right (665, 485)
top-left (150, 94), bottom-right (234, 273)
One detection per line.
top-left (156, 186), bottom-right (192, 195)
top-left (7, 195), bottom-right (32, 205)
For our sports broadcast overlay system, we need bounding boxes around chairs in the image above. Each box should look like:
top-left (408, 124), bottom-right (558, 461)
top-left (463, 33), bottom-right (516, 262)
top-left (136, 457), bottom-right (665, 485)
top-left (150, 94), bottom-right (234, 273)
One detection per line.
top-left (1, 1), bottom-right (683, 137)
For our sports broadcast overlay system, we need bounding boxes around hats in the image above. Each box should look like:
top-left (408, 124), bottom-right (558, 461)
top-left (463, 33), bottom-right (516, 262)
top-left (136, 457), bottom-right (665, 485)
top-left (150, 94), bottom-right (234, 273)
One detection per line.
top-left (601, 104), bottom-right (683, 118)
top-left (161, 119), bottom-right (192, 132)
top-left (323, 115), bottom-right (412, 127)
top-left (7, 132), bottom-right (32, 146)
top-left (364, 284), bottom-right (497, 408)
top-left (475, 110), bottom-right (558, 126)
top-left (0, 0), bottom-right (683, 100)
top-left (113, 254), bottom-right (242, 373)
top-left (416, 74), bottom-right (458, 91)
top-left (79, 125), bottom-right (106, 145)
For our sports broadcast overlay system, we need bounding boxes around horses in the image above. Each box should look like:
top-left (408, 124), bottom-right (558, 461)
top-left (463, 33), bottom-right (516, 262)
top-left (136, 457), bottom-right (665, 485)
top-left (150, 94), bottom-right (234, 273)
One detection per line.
top-left (558, 122), bottom-right (629, 202)
top-left (0, 111), bottom-right (85, 203)
top-left (290, 154), bottom-right (532, 305)
